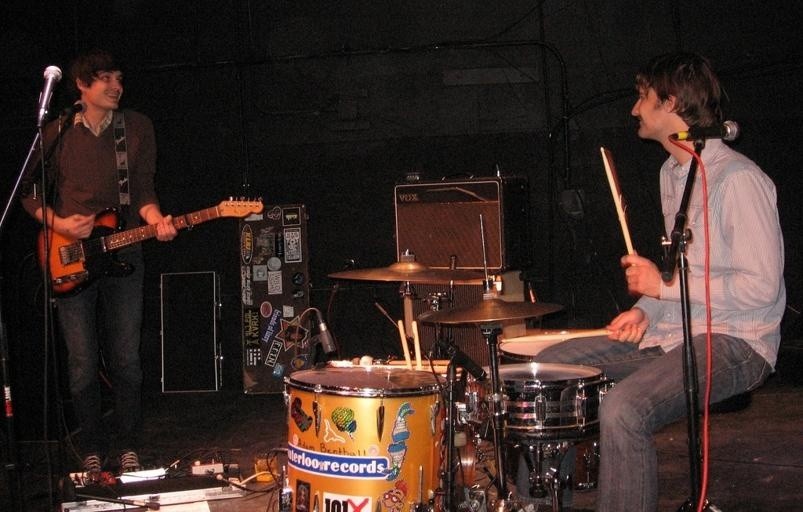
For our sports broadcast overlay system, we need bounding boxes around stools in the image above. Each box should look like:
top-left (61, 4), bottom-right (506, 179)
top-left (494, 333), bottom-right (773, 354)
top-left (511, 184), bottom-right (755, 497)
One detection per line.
top-left (676, 391), bottom-right (751, 512)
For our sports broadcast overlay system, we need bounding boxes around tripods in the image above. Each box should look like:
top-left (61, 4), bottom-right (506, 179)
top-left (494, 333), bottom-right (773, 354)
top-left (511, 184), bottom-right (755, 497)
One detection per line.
top-left (11, 122), bottom-right (166, 509)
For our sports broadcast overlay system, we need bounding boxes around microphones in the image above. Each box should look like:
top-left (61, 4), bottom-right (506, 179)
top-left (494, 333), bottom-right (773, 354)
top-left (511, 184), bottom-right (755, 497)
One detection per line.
top-left (665, 120), bottom-right (740, 143)
top-left (437, 337), bottom-right (488, 386)
top-left (37, 64), bottom-right (64, 128)
top-left (308, 312), bottom-right (338, 355)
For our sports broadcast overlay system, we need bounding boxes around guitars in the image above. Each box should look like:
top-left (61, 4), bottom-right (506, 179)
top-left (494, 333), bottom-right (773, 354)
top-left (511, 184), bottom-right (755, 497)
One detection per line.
top-left (36, 195), bottom-right (266, 300)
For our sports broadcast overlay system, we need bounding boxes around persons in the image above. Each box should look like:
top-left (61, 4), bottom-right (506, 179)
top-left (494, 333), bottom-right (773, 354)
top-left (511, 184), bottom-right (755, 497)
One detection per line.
top-left (528, 56), bottom-right (787, 510)
top-left (14, 54), bottom-right (178, 473)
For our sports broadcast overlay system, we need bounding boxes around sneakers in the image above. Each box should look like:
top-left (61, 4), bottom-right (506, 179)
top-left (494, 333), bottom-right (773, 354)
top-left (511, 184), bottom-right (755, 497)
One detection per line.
top-left (83, 453), bottom-right (102, 473)
top-left (117, 450), bottom-right (142, 473)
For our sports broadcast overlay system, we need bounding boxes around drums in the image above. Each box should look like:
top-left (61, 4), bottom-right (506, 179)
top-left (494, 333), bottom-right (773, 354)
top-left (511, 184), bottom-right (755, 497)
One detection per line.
top-left (498, 329), bottom-right (611, 363)
top-left (283, 365), bottom-right (449, 511)
top-left (467, 360), bottom-right (616, 440)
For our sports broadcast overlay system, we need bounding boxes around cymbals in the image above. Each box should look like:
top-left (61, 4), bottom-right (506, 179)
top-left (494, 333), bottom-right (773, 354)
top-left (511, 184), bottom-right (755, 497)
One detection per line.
top-left (417, 287), bottom-right (567, 325)
top-left (327, 250), bottom-right (486, 283)
